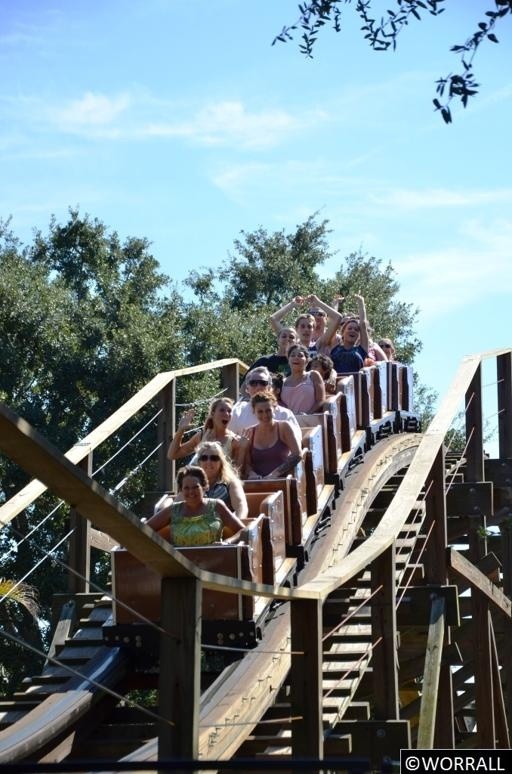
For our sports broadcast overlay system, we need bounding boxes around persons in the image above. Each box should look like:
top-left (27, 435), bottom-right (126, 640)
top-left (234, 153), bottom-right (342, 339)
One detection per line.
top-left (225, 361), bottom-right (302, 445)
top-left (165, 395), bottom-right (243, 462)
top-left (147, 464), bottom-right (247, 544)
top-left (172, 438), bottom-right (249, 520)
top-left (240, 389), bottom-right (302, 480)
top-left (239, 289), bottom-right (396, 413)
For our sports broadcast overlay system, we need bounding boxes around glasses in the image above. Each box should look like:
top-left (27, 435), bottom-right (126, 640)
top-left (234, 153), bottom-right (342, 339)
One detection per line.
top-left (198, 455), bottom-right (221, 461)
top-left (312, 312), bottom-right (327, 317)
top-left (380, 345), bottom-right (391, 348)
top-left (247, 380), bottom-right (270, 387)
